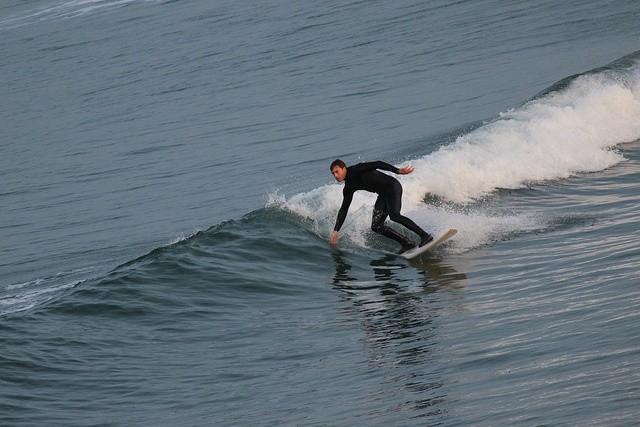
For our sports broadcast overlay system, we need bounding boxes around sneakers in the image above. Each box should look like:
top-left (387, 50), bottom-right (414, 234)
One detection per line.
top-left (418, 234), bottom-right (433, 248)
top-left (397, 242), bottom-right (416, 256)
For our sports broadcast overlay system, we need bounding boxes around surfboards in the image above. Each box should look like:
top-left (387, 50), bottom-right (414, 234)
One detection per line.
top-left (399, 227), bottom-right (458, 259)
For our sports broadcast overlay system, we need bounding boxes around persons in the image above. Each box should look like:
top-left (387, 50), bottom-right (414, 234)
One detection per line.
top-left (330, 159), bottom-right (433, 255)
